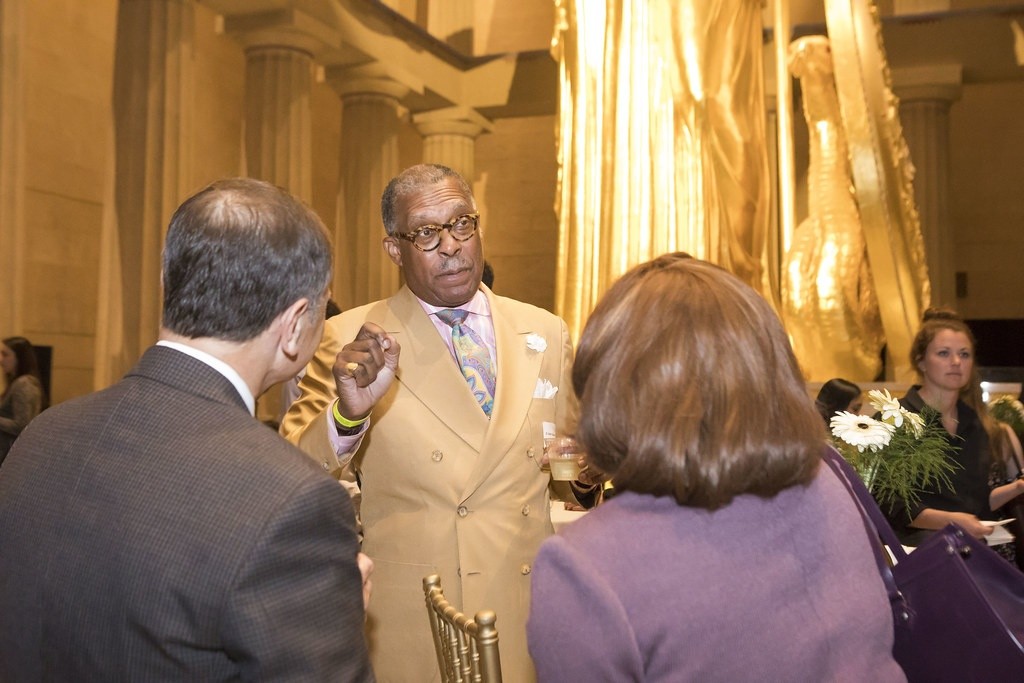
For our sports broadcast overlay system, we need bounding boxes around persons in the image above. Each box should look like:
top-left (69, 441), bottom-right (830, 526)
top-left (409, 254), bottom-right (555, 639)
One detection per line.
top-left (524, 250), bottom-right (907, 683)
top-left (0, 336), bottom-right (46, 464)
top-left (866, 305), bottom-right (1023, 571)
top-left (0, 178), bottom-right (377, 682)
top-left (282, 299), bottom-right (343, 419)
top-left (279, 162), bottom-right (608, 683)
top-left (814, 376), bottom-right (863, 426)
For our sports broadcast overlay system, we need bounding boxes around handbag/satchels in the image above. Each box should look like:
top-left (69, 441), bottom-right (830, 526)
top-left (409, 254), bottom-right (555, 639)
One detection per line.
top-left (817, 446), bottom-right (1024, 683)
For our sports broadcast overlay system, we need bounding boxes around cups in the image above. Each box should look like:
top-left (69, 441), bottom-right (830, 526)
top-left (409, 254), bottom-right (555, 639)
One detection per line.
top-left (543, 437), bottom-right (585, 483)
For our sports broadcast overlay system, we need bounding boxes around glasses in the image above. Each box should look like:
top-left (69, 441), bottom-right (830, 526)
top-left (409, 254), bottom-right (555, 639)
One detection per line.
top-left (388, 210), bottom-right (481, 252)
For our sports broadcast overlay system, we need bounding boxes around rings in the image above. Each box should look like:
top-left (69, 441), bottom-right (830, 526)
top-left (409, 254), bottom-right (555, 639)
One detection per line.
top-left (345, 362), bottom-right (359, 377)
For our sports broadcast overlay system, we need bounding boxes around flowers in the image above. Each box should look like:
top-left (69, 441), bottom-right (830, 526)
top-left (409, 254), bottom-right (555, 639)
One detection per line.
top-left (991, 395), bottom-right (1024, 425)
top-left (823, 384), bottom-right (923, 452)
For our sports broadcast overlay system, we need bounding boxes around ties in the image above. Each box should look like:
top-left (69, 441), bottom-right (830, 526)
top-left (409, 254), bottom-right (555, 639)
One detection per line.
top-left (434, 308), bottom-right (496, 419)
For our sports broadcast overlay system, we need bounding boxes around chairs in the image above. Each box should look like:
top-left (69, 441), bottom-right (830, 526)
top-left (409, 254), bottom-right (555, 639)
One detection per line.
top-left (423, 574), bottom-right (504, 683)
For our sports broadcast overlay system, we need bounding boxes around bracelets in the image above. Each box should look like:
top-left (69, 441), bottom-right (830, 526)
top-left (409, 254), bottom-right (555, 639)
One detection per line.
top-left (333, 399), bottom-right (372, 428)
